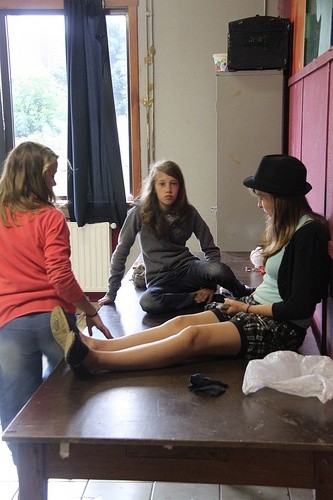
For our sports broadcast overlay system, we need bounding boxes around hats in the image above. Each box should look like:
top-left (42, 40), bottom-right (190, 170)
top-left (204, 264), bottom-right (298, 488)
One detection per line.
top-left (242, 153), bottom-right (314, 195)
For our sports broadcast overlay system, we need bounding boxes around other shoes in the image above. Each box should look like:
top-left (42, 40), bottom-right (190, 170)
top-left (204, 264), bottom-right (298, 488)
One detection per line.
top-left (63, 330), bottom-right (93, 381)
top-left (50, 304), bottom-right (81, 350)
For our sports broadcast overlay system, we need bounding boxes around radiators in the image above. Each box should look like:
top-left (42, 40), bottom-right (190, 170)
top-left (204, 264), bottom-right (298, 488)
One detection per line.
top-left (52, 222), bottom-right (119, 293)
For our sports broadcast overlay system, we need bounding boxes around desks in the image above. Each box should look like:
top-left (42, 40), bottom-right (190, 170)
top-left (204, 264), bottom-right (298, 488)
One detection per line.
top-left (0, 248), bottom-right (332, 499)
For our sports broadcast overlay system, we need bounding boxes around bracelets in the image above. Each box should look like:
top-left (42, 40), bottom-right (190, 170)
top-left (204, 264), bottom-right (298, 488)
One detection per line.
top-left (86, 313), bottom-right (98, 317)
top-left (247, 305), bottom-right (250, 312)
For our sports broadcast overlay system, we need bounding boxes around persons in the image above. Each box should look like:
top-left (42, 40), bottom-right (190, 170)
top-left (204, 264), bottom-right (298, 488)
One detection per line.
top-left (0, 141), bottom-right (112, 467)
top-left (98, 161), bottom-right (257, 315)
top-left (50, 153), bottom-right (333, 375)
